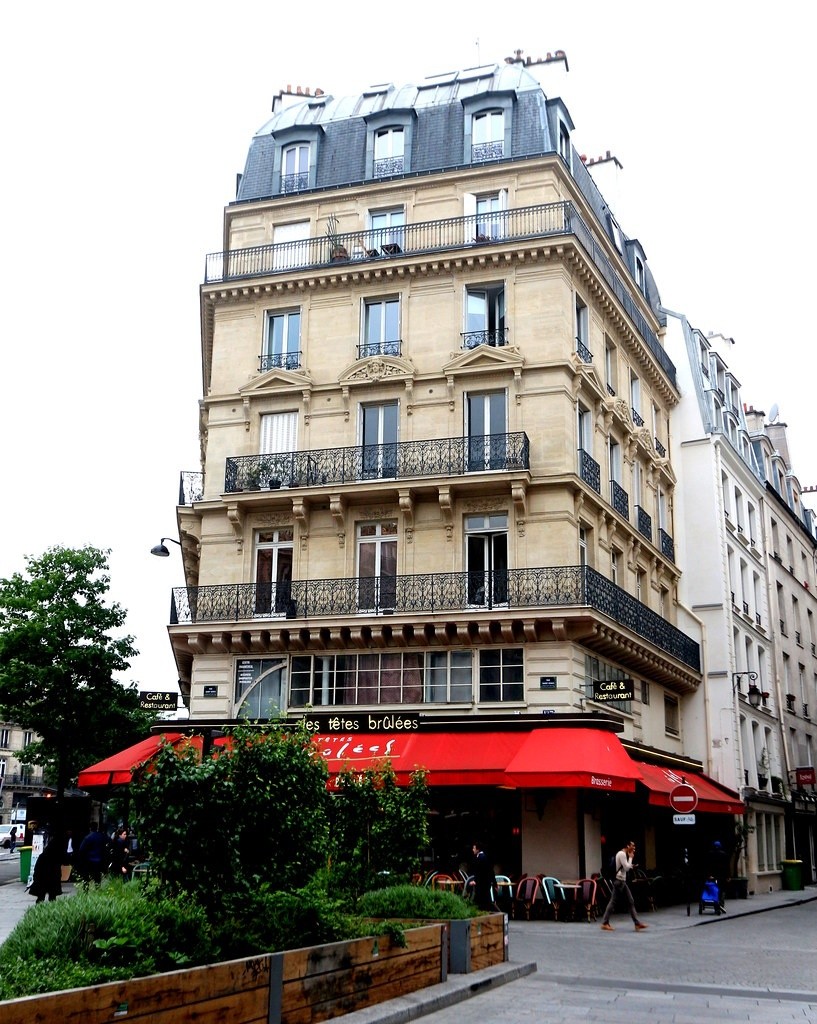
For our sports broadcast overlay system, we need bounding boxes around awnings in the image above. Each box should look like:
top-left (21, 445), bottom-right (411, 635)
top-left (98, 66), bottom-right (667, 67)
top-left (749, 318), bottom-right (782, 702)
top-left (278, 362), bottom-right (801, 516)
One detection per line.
top-left (77, 728), bottom-right (644, 792)
top-left (632, 759), bottom-right (746, 814)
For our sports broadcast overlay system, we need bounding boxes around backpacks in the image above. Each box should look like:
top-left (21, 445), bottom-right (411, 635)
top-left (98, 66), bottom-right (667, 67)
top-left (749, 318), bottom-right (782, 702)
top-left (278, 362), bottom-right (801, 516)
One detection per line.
top-left (600, 851), bottom-right (629, 881)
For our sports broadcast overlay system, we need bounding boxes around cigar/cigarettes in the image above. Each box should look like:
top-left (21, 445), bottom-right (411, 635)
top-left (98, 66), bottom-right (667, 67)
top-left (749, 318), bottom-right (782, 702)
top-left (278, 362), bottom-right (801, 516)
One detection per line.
top-left (633, 850), bottom-right (635, 851)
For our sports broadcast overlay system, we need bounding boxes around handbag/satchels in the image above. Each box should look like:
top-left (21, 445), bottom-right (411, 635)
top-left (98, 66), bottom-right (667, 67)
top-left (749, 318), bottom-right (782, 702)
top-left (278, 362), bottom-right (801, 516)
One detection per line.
top-left (701, 881), bottom-right (719, 902)
top-left (627, 864), bottom-right (647, 880)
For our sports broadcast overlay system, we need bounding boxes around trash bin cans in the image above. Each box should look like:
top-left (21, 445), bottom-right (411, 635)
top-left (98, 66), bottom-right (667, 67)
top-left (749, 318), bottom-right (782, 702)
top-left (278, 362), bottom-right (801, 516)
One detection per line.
top-left (17, 845), bottom-right (32, 884)
top-left (780, 859), bottom-right (803, 891)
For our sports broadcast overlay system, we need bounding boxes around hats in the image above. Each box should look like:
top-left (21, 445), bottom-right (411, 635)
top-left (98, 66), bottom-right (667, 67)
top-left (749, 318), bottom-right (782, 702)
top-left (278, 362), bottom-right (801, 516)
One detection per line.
top-left (709, 841), bottom-right (723, 848)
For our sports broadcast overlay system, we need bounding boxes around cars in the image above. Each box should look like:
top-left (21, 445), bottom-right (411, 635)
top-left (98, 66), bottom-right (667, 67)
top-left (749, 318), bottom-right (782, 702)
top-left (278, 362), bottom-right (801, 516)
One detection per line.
top-left (0, 824), bottom-right (25, 848)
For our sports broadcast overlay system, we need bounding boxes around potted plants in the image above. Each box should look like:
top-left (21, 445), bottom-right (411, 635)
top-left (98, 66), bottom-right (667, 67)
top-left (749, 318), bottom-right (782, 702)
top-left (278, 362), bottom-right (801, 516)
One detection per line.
top-left (723, 822), bottom-right (754, 899)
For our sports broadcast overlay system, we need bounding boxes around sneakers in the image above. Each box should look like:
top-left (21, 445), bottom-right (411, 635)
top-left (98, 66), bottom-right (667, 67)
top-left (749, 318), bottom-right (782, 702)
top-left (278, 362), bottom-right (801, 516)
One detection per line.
top-left (635, 924), bottom-right (649, 930)
top-left (600, 923), bottom-right (615, 930)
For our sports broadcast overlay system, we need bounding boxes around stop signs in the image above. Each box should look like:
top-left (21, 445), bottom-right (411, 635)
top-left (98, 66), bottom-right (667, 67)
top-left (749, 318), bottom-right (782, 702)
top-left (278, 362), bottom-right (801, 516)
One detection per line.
top-left (670, 784), bottom-right (698, 814)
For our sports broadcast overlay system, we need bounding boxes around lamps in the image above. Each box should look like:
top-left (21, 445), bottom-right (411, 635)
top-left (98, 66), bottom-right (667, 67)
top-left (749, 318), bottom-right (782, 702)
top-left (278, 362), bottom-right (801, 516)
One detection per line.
top-left (732, 672), bottom-right (762, 710)
top-left (150, 537), bottom-right (181, 556)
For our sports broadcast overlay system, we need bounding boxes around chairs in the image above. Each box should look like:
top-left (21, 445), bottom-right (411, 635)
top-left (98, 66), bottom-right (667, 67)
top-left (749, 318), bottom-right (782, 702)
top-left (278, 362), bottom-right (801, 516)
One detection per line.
top-left (408, 870), bottom-right (661, 920)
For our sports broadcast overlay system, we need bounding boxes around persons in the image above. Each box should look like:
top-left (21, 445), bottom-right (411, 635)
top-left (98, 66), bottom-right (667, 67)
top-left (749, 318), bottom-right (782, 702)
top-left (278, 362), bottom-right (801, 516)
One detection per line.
top-left (468, 840), bottom-right (498, 912)
top-left (600, 840), bottom-right (648, 931)
top-left (26, 839), bottom-right (69, 904)
top-left (78, 822), bottom-right (114, 890)
top-left (112, 829), bottom-right (130, 882)
top-left (10, 829), bottom-right (17, 853)
top-left (706, 841), bottom-right (730, 914)
top-left (101, 822), bottom-right (135, 840)
top-left (64, 829), bottom-right (80, 868)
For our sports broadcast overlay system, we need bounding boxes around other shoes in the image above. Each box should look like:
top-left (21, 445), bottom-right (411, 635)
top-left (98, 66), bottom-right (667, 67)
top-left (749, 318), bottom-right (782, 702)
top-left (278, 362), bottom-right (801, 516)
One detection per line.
top-left (720, 906), bottom-right (727, 913)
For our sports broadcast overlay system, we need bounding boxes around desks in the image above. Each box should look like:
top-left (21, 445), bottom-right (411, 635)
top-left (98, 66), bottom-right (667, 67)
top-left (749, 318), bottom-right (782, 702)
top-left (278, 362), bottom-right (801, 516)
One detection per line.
top-left (437, 881), bottom-right (464, 894)
top-left (493, 881), bottom-right (518, 920)
top-left (555, 883), bottom-right (582, 920)
